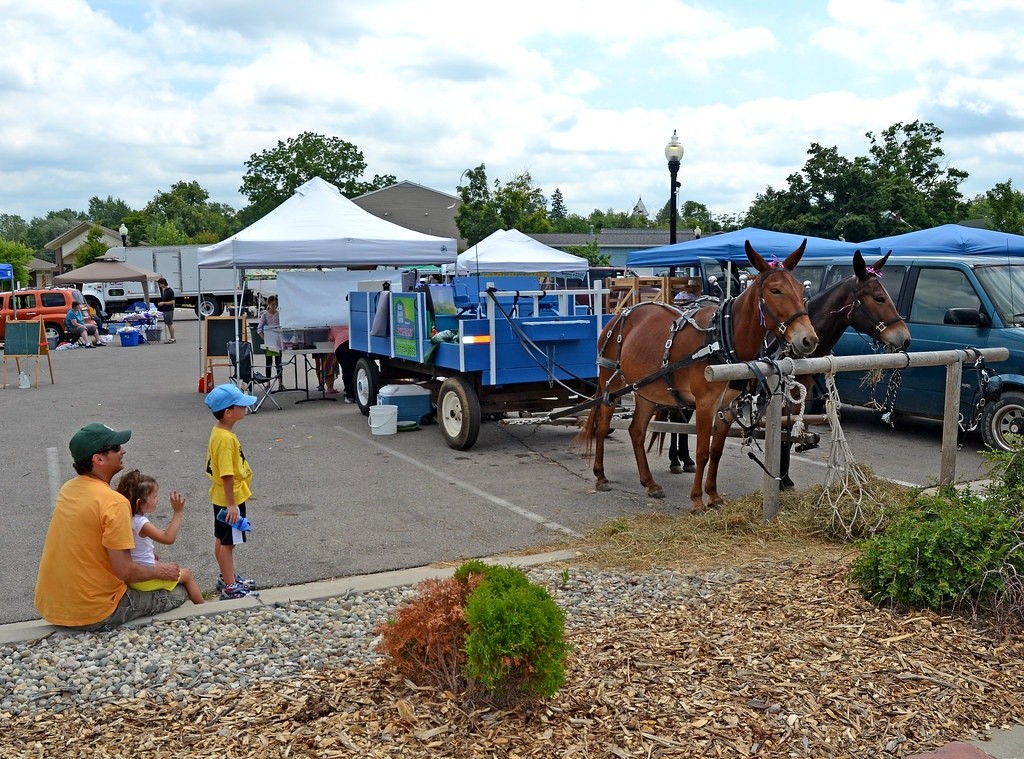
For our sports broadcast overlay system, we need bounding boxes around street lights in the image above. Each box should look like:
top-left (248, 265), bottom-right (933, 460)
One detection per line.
top-left (119, 223), bottom-right (128, 247)
top-left (665, 127), bottom-right (685, 277)
top-left (732, 210), bottom-right (745, 229)
top-left (693, 225), bottom-right (701, 276)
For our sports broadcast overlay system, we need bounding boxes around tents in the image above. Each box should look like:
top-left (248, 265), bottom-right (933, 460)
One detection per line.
top-left (399, 265), bottom-right (442, 283)
top-left (441, 228), bottom-right (591, 316)
top-left (625, 227), bottom-right (883, 299)
top-left (0, 263), bottom-right (16, 319)
top-left (198, 176), bottom-right (457, 391)
top-left (857, 224), bottom-right (1024, 256)
top-left (53, 254), bottom-right (164, 311)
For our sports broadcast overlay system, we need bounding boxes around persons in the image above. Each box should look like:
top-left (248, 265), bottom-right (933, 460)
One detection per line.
top-left (256, 292), bottom-right (264, 304)
top-left (256, 297), bottom-right (286, 392)
top-left (158, 278), bottom-right (176, 344)
top-left (328, 326), bottom-right (367, 404)
top-left (34, 423), bottom-right (188, 632)
top-left (88, 302), bottom-right (106, 335)
top-left (320, 353), bottom-right (339, 394)
top-left (114, 467), bottom-right (206, 604)
top-left (311, 353), bottom-right (324, 388)
top-left (66, 301), bottom-right (107, 348)
top-left (205, 384), bottom-right (257, 601)
top-left (673, 279), bottom-right (702, 299)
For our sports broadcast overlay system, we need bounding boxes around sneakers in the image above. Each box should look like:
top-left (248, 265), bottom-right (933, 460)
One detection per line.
top-left (216, 573), bottom-right (256, 591)
top-left (219, 583), bottom-right (259, 601)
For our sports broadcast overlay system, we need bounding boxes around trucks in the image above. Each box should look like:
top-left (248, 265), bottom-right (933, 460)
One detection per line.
top-left (70, 245), bottom-right (246, 321)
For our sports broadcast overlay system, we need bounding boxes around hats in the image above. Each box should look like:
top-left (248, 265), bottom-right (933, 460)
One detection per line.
top-left (204, 383), bottom-right (258, 412)
top-left (71, 301), bottom-right (82, 309)
top-left (69, 422), bottom-right (131, 462)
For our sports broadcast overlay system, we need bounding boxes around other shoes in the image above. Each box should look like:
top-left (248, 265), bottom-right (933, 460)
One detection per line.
top-left (265, 385), bottom-right (271, 393)
top-left (279, 386), bottom-right (286, 391)
top-left (318, 384), bottom-right (325, 391)
top-left (168, 338), bottom-right (176, 344)
top-left (326, 389), bottom-right (339, 394)
top-left (85, 345), bottom-right (96, 348)
top-left (95, 343), bottom-right (107, 346)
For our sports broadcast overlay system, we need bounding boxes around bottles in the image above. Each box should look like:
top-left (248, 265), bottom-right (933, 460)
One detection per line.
top-left (217, 509), bottom-right (252, 533)
top-left (432, 326), bottom-right (437, 336)
top-left (199, 374), bottom-right (204, 393)
top-left (292, 331), bottom-right (299, 350)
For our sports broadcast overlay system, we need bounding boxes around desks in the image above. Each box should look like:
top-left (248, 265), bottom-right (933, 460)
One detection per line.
top-left (124, 317), bottom-right (160, 345)
top-left (260, 341), bottom-right (341, 405)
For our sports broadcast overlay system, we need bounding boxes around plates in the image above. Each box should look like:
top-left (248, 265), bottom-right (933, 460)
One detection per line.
top-left (397, 421), bottom-right (419, 430)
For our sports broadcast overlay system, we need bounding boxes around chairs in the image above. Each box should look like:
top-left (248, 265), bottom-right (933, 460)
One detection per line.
top-left (62, 320), bottom-right (86, 348)
top-left (225, 341), bottom-right (282, 415)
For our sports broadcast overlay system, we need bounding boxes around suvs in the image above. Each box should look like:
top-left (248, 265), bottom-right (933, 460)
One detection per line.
top-left (0, 287), bottom-right (97, 350)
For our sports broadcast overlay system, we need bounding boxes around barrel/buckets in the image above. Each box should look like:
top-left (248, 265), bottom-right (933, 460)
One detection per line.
top-left (368, 405), bottom-right (398, 436)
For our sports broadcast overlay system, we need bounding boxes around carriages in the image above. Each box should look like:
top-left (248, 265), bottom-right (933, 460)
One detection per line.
top-left (345, 239), bottom-right (912, 516)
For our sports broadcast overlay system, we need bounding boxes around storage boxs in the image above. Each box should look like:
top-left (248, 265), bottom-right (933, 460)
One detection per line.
top-left (46, 335), bottom-right (59, 350)
top-left (145, 329), bottom-right (162, 341)
top-left (417, 282), bottom-right (455, 315)
top-left (119, 330), bottom-right (139, 347)
top-left (375, 387), bottom-right (432, 424)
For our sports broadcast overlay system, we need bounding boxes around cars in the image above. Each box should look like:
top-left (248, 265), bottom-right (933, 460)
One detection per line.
top-left (760, 253), bottom-right (1024, 454)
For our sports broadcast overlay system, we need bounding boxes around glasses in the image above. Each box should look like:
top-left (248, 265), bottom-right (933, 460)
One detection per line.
top-left (98, 444), bottom-right (121, 455)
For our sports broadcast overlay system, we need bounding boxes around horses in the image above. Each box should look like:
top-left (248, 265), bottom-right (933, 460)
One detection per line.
top-left (645, 249), bottom-right (912, 490)
top-left (567, 236), bottom-right (820, 513)
